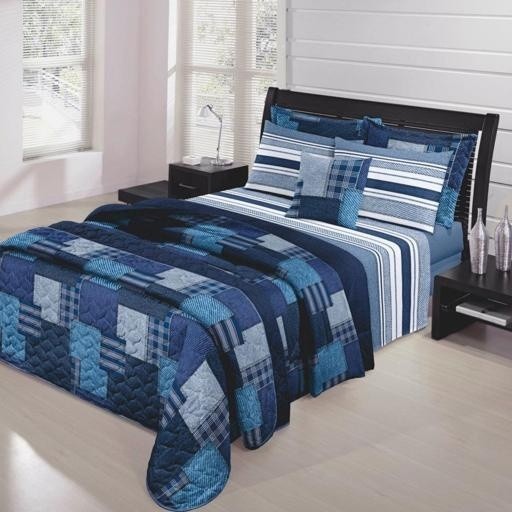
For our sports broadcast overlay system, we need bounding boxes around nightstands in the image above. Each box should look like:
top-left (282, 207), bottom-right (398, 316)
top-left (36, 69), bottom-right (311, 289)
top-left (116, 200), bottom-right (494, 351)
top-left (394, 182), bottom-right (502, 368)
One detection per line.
top-left (168, 157), bottom-right (248, 198)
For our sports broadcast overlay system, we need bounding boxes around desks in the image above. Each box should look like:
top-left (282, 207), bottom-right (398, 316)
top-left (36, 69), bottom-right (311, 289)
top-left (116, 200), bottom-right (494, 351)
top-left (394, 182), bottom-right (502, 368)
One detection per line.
top-left (431, 255), bottom-right (512, 340)
top-left (119, 180), bottom-right (168, 205)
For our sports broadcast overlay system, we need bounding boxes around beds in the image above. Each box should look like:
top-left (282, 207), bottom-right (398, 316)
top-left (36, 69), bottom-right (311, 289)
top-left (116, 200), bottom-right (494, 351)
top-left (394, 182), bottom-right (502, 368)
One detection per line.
top-left (0, 87), bottom-right (499, 512)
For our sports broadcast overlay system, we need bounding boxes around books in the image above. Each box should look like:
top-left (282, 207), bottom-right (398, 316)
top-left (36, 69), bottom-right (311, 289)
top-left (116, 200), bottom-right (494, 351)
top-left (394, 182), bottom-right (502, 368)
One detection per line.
top-left (456, 299), bottom-right (511, 328)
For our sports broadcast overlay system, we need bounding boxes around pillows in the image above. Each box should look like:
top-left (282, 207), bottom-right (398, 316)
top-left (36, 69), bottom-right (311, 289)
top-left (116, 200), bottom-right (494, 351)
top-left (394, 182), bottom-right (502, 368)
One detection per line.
top-left (242, 105), bottom-right (477, 231)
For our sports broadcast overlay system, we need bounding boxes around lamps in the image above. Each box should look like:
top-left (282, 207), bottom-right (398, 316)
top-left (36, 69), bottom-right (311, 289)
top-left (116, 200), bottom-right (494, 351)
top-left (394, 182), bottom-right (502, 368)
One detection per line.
top-left (198, 103), bottom-right (232, 166)
